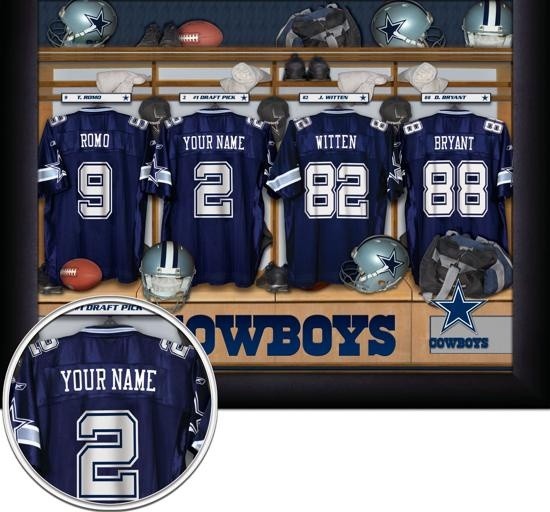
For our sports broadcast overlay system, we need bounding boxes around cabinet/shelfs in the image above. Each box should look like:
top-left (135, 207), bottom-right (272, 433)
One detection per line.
top-left (39, 44), bottom-right (512, 367)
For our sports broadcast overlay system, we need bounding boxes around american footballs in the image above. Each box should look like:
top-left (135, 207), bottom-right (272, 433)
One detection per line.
top-left (59, 259), bottom-right (102, 290)
top-left (178, 21), bottom-right (224, 47)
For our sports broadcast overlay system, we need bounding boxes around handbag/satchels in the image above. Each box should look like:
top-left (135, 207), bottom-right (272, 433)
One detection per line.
top-left (418, 230), bottom-right (513, 307)
top-left (275, 3), bottom-right (361, 48)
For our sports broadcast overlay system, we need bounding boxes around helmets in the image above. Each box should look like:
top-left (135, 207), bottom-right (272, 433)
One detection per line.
top-left (461, 0), bottom-right (513, 49)
top-left (370, 0), bottom-right (433, 48)
top-left (350, 234), bottom-right (409, 294)
top-left (138, 240), bottom-right (197, 302)
top-left (57, 0), bottom-right (118, 47)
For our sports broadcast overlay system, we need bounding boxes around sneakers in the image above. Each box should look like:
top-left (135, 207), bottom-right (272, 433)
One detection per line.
top-left (135, 22), bottom-right (182, 47)
top-left (256, 261), bottom-right (289, 293)
top-left (283, 54), bottom-right (331, 81)
